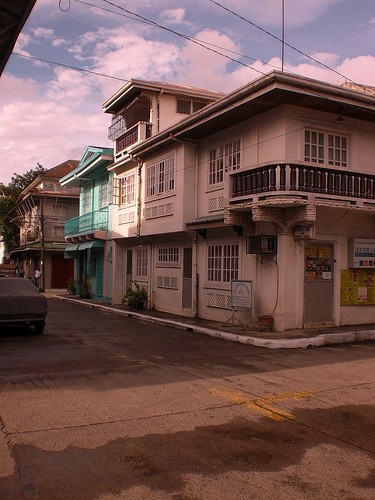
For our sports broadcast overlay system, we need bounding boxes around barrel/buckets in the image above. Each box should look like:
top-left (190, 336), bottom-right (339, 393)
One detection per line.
top-left (257, 315), bottom-right (273, 331)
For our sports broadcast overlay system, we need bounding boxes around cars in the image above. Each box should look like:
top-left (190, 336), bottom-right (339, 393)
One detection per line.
top-left (0, 277), bottom-right (49, 335)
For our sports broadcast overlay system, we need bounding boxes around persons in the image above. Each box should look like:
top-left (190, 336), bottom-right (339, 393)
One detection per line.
top-left (18, 267), bottom-right (25, 277)
top-left (15, 266), bottom-right (19, 277)
top-left (35, 267), bottom-right (41, 287)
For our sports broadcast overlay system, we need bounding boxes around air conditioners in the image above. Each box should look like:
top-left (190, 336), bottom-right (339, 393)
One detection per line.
top-left (247, 234), bottom-right (277, 255)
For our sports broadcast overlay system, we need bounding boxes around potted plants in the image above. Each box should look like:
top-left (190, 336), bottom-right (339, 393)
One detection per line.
top-left (120, 280), bottom-right (148, 309)
top-left (67, 277), bottom-right (91, 299)
